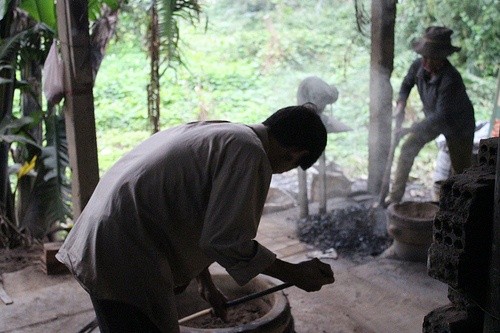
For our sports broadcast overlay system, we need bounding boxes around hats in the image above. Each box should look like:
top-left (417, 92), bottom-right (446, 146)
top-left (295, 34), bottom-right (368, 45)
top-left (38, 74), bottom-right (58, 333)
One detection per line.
top-left (408, 27), bottom-right (462, 58)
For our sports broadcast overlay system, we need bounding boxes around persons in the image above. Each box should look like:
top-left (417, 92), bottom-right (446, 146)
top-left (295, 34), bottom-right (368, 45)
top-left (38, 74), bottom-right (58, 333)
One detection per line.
top-left (55, 106), bottom-right (335, 333)
top-left (374, 26), bottom-right (476, 208)
